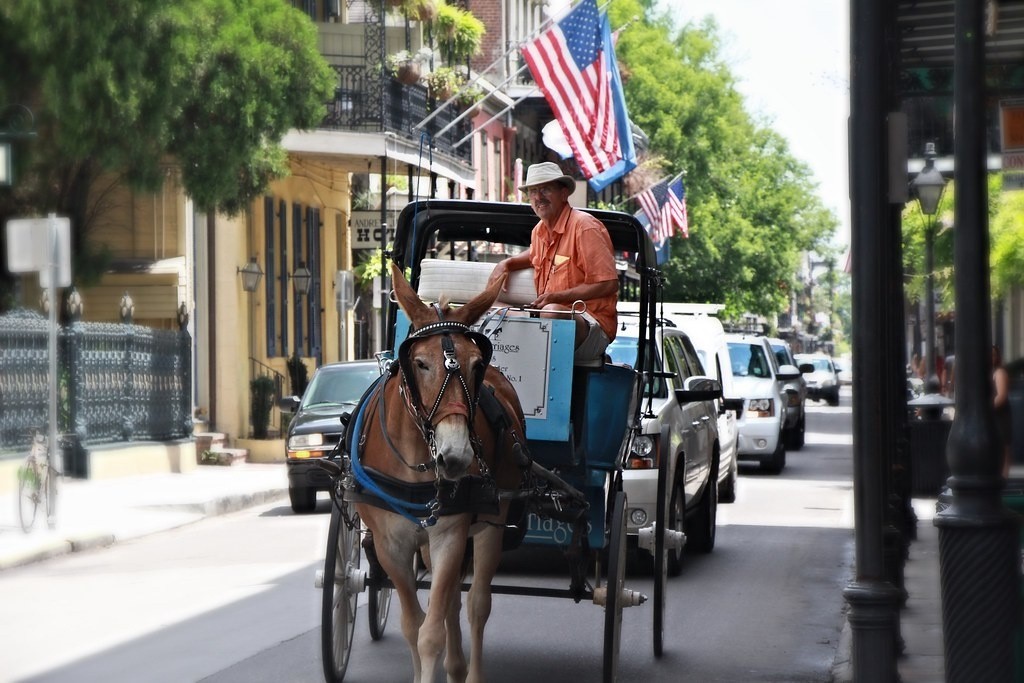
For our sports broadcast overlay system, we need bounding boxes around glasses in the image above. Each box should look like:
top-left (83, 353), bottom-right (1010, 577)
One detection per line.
top-left (524, 187), bottom-right (562, 199)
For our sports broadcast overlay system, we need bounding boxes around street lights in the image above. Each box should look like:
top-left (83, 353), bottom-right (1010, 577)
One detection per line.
top-left (909, 140), bottom-right (956, 420)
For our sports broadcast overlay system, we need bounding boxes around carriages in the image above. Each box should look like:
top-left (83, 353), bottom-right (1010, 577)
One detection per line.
top-left (309, 196), bottom-right (691, 683)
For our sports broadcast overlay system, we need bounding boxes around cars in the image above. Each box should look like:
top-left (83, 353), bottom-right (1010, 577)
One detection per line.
top-left (793, 352), bottom-right (842, 407)
top-left (723, 330), bottom-right (801, 477)
top-left (760, 335), bottom-right (816, 453)
top-left (275, 357), bottom-right (394, 514)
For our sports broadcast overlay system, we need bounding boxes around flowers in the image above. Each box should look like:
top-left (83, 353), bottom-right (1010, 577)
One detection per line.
top-left (384, 46), bottom-right (433, 78)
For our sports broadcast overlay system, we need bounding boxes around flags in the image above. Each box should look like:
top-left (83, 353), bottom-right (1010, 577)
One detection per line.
top-left (669, 180), bottom-right (688, 238)
top-left (589, 8), bottom-right (638, 192)
top-left (638, 182), bottom-right (675, 240)
top-left (520, 0), bottom-right (622, 181)
top-left (634, 211), bottom-right (670, 264)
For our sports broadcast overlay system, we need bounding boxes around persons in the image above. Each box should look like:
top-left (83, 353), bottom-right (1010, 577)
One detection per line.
top-left (487, 163), bottom-right (618, 361)
top-left (913, 347), bottom-right (1024, 477)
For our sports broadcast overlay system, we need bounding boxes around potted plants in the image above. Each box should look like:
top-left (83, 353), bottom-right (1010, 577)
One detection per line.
top-left (456, 77), bottom-right (484, 118)
top-left (428, 68), bottom-right (456, 108)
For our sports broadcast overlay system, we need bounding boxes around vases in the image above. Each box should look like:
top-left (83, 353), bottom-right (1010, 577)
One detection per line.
top-left (398, 63), bottom-right (421, 84)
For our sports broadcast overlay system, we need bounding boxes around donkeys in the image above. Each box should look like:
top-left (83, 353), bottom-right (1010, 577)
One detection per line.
top-left (352, 262), bottom-right (525, 683)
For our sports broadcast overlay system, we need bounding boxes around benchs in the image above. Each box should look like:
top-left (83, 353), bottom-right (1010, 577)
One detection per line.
top-left (413, 255), bottom-right (608, 372)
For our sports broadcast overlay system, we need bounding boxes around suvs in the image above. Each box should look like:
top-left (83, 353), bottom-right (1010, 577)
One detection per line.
top-left (604, 311), bottom-right (723, 579)
top-left (614, 299), bottom-right (744, 505)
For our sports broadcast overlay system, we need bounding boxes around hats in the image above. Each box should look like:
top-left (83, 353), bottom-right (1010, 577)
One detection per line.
top-left (519, 162), bottom-right (575, 196)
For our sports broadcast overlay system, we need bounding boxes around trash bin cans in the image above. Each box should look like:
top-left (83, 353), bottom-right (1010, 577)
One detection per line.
top-left (909, 393), bottom-right (955, 499)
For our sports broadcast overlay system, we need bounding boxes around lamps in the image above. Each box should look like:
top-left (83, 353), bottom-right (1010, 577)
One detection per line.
top-left (236, 251), bottom-right (265, 295)
top-left (288, 260), bottom-right (313, 296)
